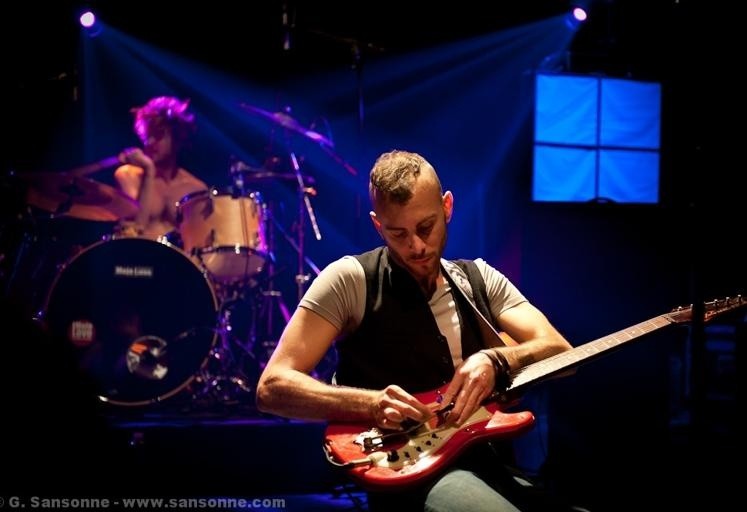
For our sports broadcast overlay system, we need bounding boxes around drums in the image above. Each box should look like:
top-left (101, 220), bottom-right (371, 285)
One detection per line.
top-left (175, 186), bottom-right (270, 288)
top-left (35, 233), bottom-right (220, 405)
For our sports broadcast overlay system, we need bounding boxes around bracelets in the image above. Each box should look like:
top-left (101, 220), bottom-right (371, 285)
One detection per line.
top-left (478, 347), bottom-right (513, 395)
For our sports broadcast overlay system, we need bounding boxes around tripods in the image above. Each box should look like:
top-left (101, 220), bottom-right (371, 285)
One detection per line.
top-left (194, 225), bottom-right (293, 404)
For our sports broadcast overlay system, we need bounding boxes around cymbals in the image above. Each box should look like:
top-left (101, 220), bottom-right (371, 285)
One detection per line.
top-left (20, 169), bottom-right (142, 221)
top-left (242, 172), bottom-right (316, 186)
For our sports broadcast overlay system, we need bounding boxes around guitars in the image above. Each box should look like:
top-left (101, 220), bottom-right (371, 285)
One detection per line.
top-left (323, 294), bottom-right (747, 494)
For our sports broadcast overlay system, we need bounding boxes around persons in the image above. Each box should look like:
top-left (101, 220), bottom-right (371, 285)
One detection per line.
top-left (116, 96), bottom-right (209, 241)
top-left (256, 149), bottom-right (577, 512)
